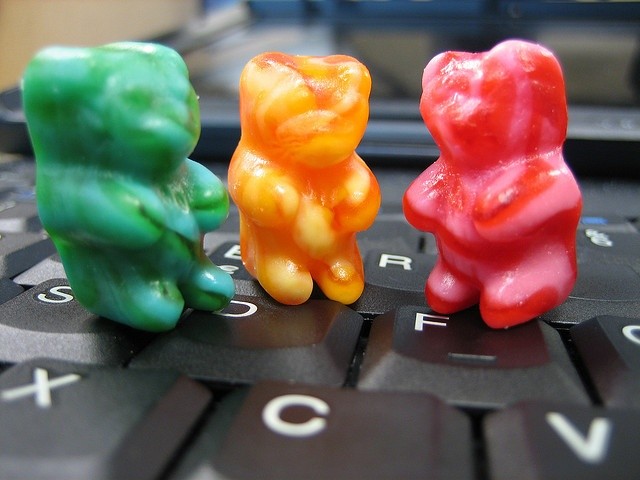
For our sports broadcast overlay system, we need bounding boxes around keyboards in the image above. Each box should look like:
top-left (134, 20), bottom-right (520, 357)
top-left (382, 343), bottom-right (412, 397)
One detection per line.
top-left (1, 141), bottom-right (639, 474)
